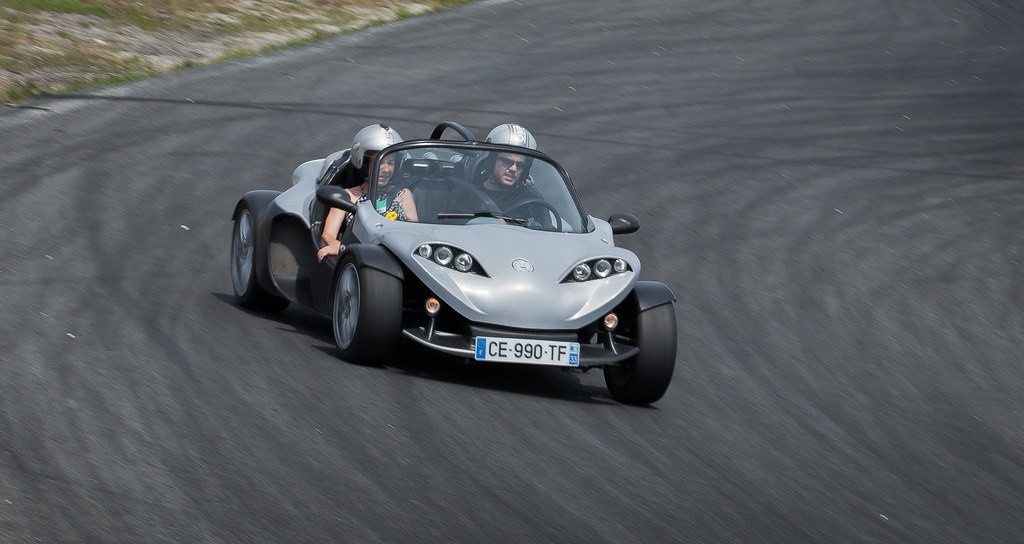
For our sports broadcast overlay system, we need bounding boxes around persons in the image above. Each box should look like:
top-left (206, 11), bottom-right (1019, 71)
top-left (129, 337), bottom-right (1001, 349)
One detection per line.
top-left (448, 124), bottom-right (556, 231)
top-left (316, 123), bottom-right (419, 260)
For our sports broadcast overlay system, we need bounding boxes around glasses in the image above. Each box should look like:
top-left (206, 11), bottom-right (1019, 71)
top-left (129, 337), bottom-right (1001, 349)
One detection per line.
top-left (495, 156), bottom-right (527, 170)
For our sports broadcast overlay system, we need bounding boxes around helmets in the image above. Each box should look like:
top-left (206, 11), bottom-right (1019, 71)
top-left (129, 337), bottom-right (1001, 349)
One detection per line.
top-left (349, 123), bottom-right (408, 170)
top-left (484, 123), bottom-right (537, 158)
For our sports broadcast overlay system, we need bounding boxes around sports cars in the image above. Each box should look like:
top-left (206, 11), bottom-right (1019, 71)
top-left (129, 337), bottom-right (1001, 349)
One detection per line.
top-left (230, 122), bottom-right (678, 404)
